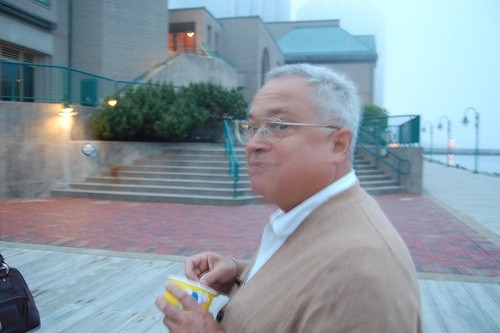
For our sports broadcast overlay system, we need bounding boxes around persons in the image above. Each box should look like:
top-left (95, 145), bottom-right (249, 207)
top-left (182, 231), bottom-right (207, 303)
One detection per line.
top-left (154, 63), bottom-right (424, 333)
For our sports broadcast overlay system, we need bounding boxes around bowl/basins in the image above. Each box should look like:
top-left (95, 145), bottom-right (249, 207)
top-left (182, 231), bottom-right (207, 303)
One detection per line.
top-left (164, 276), bottom-right (217, 314)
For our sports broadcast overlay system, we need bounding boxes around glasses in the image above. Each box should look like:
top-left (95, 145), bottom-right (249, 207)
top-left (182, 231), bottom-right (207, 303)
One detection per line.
top-left (234, 116), bottom-right (340, 145)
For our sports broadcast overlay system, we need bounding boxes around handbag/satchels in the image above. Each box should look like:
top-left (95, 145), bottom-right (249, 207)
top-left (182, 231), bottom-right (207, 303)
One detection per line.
top-left (0, 255), bottom-right (40, 333)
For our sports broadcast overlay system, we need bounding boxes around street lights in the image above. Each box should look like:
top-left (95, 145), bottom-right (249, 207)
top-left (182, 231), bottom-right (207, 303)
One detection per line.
top-left (421, 120), bottom-right (435, 159)
top-left (438, 116), bottom-right (452, 166)
top-left (461, 107), bottom-right (480, 175)
